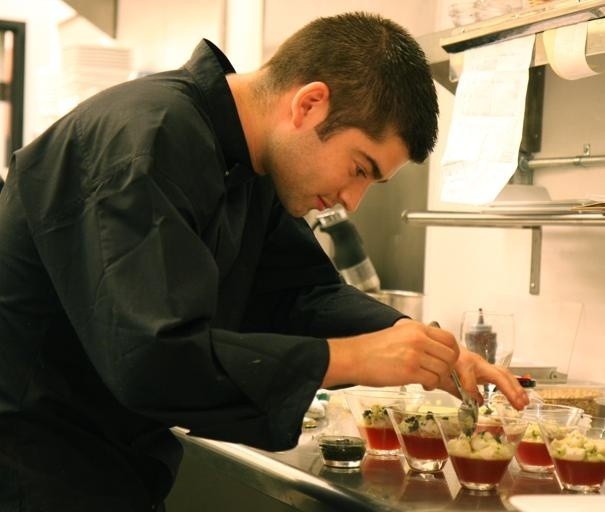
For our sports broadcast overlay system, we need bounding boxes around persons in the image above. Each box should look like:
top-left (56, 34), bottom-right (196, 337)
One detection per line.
top-left (2, 12), bottom-right (531, 512)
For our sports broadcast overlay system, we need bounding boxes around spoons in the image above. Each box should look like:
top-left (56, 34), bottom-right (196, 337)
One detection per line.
top-left (430, 321), bottom-right (480, 437)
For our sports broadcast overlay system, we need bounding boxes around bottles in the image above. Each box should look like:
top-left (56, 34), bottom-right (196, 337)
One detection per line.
top-left (464, 306), bottom-right (498, 367)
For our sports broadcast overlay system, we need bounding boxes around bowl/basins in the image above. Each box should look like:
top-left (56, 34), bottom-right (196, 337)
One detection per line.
top-left (310, 432), bottom-right (369, 469)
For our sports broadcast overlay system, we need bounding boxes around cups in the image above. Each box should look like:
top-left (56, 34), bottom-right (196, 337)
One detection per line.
top-left (347, 384), bottom-right (605, 495)
